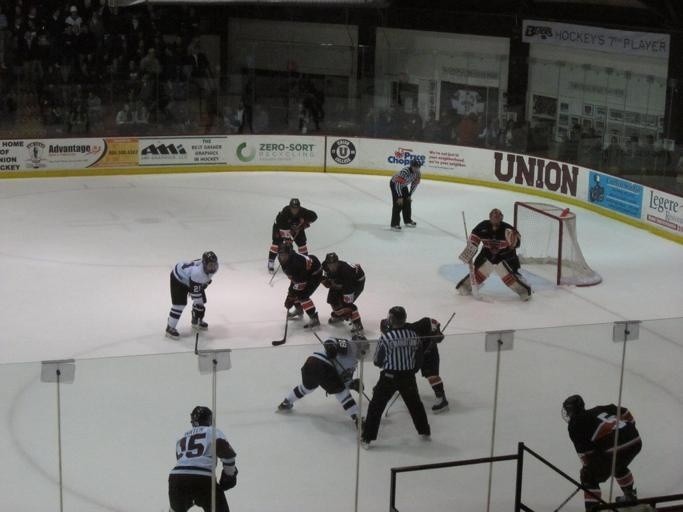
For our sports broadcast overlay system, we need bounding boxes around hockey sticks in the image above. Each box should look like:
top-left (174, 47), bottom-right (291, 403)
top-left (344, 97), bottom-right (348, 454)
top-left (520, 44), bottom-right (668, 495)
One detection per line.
top-left (195, 318), bottom-right (207, 358)
top-left (462, 211), bottom-right (493, 302)
top-left (385, 313), bottom-right (456, 417)
top-left (272, 308), bottom-right (289, 346)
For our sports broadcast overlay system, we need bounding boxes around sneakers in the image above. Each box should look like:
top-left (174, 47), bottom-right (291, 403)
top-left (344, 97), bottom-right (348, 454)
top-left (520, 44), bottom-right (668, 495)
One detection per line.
top-left (267, 261), bottom-right (275, 270)
top-left (288, 310), bottom-right (303, 317)
top-left (304, 319), bottom-right (319, 328)
top-left (191, 319), bottom-right (208, 328)
top-left (279, 399), bottom-right (293, 410)
top-left (327, 316), bottom-right (340, 322)
top-left (166, 326), bottom-right (180, 336)
top-left (391, 221), bottom-right (416, 229)
top-left (350, 325), bottom-right (362, 331)
top-left (616, 490), bottom-right (637, 502)
top-left (432, 398), bottom-right (448, 410)
top-left (357, 420), bottom-right (371, 443)
top-left (419, 425), bottom-right (430, 434)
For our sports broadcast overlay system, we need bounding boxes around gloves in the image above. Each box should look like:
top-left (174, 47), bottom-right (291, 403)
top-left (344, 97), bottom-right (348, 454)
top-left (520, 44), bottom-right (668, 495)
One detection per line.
top-left (323, 340), bottom-right (337, 358)
top-left (219, 467), bottom-right (237, 490)
top-left (285, 294), bottom-right (295, 309)
top-left (350, 378), bottom-right (364, 392)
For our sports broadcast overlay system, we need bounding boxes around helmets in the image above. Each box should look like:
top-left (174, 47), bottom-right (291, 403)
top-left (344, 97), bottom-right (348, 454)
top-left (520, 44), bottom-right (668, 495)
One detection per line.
top-left (203, 251), bottom-right (218, 273)
top-left (278, 239), bottom-right (292, 256)
top-left (290, 198), bottom-right (300, 214)
top-left (561, 394), bottom-right (585, 422)
top-left (389, 306), bottom-right (406, 329)
top-left (325, 253), bottom-right (339, 265)
top-left (491, 209), bottom-right (503, 226)
top-left (410, 159), bottom-right (420, 170)
top-left (190, 406), bottom-right (214, 427)
top-left (352, 335), bottom-right (369, 356)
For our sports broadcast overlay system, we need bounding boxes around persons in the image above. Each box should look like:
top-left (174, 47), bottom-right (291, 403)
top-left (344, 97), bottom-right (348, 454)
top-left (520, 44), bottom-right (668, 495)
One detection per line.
top-left (163, 251), bottom-right (220, 336)
top-left (390, 156), bottom-right (421, 227)
top-left (274, 244), bottom-right (323, 330)
top-left (169, 406), bottom-right (238, 512)
top-left (456, 208), bottom-right (534, 301)
top-left (267, 197), bottom-right (319, 274)
top-left (561, 394), bottom-right (642, 512)
top-left (276, 306), bottom-right (459, 447)
top-left (0, 0), bottom-right (682, 198)
top-left (316, 252), bottom-right (365, 342)
